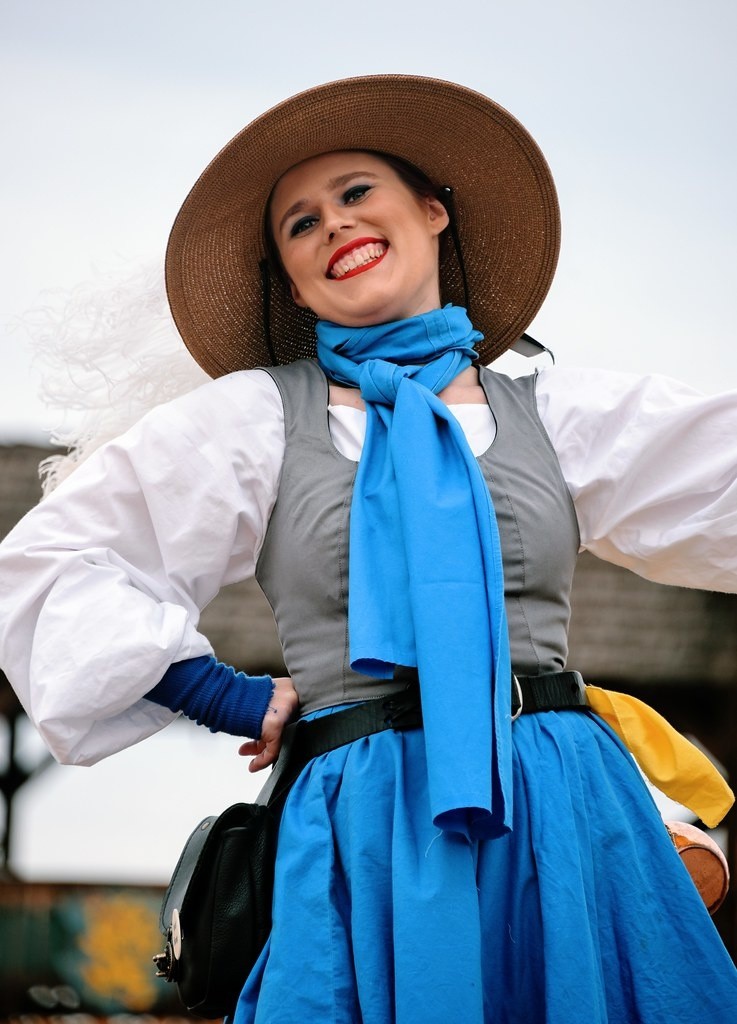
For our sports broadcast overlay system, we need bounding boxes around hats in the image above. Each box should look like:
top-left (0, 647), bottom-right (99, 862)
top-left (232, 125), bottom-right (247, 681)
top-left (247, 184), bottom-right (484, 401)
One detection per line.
top-left (163, 74), bottom-right (561, 380)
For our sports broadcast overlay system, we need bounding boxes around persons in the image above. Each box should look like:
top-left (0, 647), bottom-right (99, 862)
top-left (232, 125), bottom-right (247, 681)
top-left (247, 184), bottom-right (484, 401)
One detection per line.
top-left (0, 74), bottom-right (737, 1024)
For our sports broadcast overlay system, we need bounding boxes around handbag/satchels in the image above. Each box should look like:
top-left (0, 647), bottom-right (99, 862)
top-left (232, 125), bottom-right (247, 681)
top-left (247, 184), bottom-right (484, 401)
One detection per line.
top-left (151, 719), bottom-right (307, 1021)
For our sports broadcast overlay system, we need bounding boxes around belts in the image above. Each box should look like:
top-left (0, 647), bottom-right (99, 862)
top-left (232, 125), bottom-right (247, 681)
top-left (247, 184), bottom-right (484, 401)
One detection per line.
top-left (288, 670), bottom-right (589, 769)
